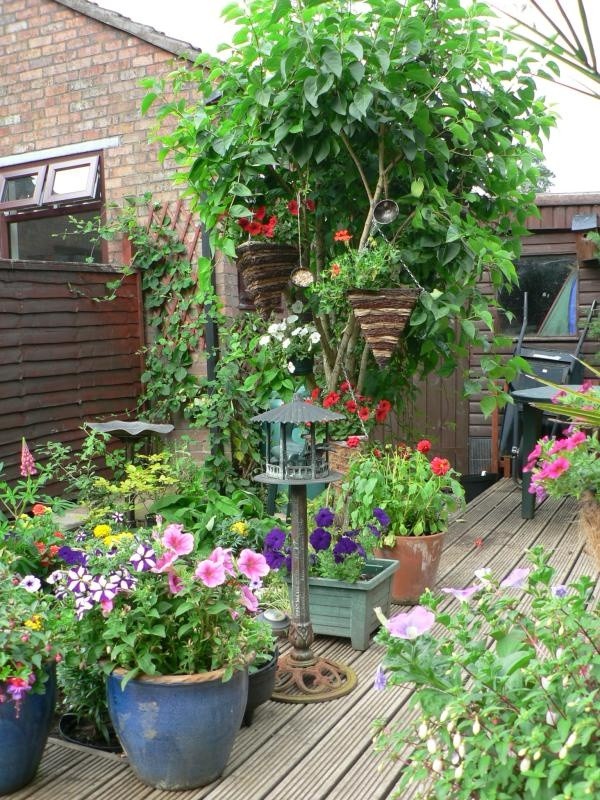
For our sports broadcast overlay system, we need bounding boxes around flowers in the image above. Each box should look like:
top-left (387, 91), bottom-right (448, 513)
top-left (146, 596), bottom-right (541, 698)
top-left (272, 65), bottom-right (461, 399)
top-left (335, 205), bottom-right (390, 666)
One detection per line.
top-left (0, 188), bottom-right (600, 800)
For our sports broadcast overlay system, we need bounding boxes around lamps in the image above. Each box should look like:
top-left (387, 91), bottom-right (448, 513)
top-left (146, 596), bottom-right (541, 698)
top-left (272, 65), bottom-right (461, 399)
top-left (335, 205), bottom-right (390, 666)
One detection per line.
top-left (250, 392), bottom-right (348, 479)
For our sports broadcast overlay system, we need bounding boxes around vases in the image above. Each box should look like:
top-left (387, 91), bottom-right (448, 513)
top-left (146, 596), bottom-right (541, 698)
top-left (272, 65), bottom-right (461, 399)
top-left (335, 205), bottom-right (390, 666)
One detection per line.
top-left (0, 662), bottom-right (57, 793)
top-left (290, 353), bottom-right (314, 375)
top-left (461, 472), bottom-right (498, 503)
top-left (239, 646), bottom-right (278, 727)
top-left (59, 709), bottom-right (123, 754)
top-left (371, 528), bottom-right (446, 606)
top-left (344, 290), bottom-right (422, 370)
top-left (284, 557), bottom-right (399, 652)
top-left (101, 659), bottom-right (248, 790)
top-left (240, 242), bottom-right (305, 323)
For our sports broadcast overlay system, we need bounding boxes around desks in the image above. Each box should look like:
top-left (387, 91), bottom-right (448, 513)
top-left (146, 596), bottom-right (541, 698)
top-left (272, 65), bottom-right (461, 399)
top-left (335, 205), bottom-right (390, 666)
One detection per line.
top-left (513, 385), bottom-right (600, 519)
